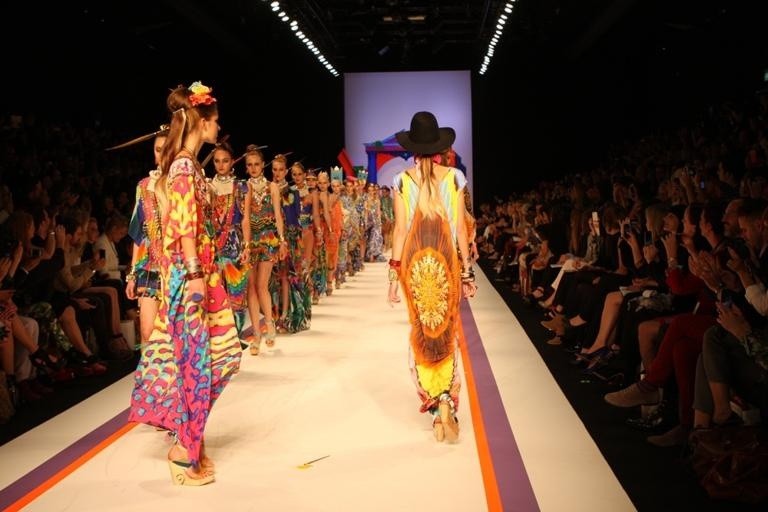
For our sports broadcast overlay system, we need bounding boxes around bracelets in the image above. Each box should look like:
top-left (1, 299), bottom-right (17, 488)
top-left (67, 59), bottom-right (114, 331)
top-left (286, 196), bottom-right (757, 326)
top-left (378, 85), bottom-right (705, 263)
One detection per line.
top-left (461, 263), bottom-right (475, 283)
top-left (389, 259), bottom-right (401, 280)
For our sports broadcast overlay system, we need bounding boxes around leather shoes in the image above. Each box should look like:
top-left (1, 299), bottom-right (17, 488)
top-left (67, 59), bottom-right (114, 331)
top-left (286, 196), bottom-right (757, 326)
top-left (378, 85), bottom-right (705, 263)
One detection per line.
top-left (604, 383), bottom-right (692, 446)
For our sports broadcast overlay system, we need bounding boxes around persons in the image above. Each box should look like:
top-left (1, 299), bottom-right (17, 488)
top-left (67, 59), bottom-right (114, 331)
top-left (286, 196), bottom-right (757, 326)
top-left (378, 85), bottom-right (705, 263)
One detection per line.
top-left (389, 112), bottom-right (477, 443)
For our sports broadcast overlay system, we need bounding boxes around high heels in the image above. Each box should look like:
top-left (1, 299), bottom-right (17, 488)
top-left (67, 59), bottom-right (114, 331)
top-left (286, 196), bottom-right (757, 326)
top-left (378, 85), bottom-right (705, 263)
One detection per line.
top-left (541, 317), bottom-right (564, 337)
top-left (250, 320), bottom-right (276, 355)
top-left (169, 443), bottom-right (215, 486)
top-left (29, 347), bottom-right (66, 370)
top-left (574, 346), bottom-right (604, 372)
top-left (434, 401), bottom-right (458, 443)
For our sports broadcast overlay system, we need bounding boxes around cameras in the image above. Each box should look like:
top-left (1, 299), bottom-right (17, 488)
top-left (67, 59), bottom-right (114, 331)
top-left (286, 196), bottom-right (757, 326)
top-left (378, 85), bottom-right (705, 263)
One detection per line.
top-left (681, 167), bottom-right (688, 178)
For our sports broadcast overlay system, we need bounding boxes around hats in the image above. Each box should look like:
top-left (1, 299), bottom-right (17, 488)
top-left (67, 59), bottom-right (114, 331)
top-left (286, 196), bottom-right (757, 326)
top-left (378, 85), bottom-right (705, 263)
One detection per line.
top-left (395, 112), bottom-right (455, 155)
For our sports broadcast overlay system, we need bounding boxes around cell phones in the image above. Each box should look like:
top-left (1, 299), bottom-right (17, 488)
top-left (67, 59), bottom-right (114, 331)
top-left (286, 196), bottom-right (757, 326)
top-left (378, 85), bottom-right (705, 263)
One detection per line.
top-left (624, 224), bottom-right (631, 239)
top-left (720, 287), bottom-right (730, 310)
